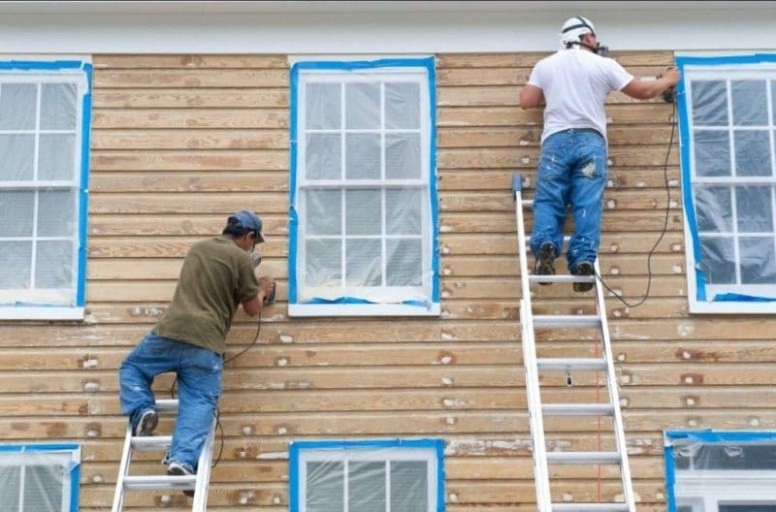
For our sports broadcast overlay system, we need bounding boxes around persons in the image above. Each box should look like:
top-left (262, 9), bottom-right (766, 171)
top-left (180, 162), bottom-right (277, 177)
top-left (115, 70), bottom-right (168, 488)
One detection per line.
top-left (119, 210), bottom-right (277, 497)
top-left (520, 16), bottom-right (682, 291)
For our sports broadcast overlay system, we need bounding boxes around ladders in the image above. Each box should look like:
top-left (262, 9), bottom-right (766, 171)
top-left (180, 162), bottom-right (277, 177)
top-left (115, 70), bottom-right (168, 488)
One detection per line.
top-left (110, 399), bottom-right (216, 512)
top-left (511, 176), bottom-right (636, 512)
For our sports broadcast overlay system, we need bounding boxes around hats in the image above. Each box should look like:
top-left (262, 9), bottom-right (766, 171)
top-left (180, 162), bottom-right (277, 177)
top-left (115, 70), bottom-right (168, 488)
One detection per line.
top-left (227, 212), bottom-right (265, 244)
top-left (561, 15), bottom-right (596, 42)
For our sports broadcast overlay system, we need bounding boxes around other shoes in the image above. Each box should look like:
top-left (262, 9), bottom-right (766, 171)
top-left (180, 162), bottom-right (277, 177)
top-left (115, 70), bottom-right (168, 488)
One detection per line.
top-left (573, 259), bottom-right (593, 292)
top-left (167, 463), bottom-right (194, 496)
top-left (535, 244), bottom-right (556, 285)
top-left (135, 410), bottom-right (158, 435)
